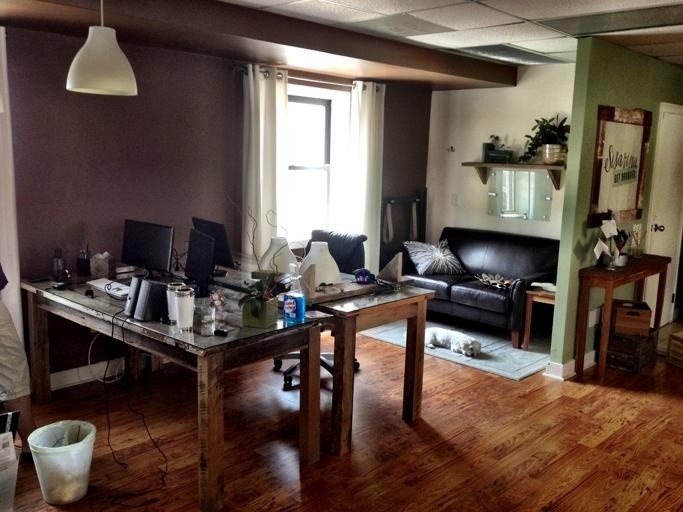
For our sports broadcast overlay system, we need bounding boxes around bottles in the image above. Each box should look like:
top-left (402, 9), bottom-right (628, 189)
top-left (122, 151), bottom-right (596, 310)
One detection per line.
top-left (284, 263), bottom-right (305, 325)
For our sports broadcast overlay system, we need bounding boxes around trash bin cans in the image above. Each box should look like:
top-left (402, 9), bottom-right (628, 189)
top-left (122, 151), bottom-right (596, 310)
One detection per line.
top-left (28, 418), bottom-right (97, 507)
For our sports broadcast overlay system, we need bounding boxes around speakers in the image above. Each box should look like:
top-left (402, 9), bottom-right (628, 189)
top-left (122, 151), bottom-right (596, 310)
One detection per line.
top-left (134, 279), bottom-right (171, 324)
top-left (124, 276), bottom-right (143, 318)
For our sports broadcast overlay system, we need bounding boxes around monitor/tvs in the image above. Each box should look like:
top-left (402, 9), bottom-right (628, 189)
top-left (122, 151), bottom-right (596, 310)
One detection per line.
top-left (192, 216), bottom-right (234, 276)
top-left (185, 228), bottom-right (216, 298)
top-left (122, 219), bottom-right (174, 279)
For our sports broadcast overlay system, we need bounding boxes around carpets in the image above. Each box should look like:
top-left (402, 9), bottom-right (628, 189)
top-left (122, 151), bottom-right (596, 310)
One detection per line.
top-left (651, 321), bottom-right (680, 356)
top-left (357, 317), bottom-right (551, 381)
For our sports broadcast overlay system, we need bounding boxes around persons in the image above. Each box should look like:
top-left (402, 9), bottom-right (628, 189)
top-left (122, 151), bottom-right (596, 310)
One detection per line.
top-left (1, 260), bottom-right (40, 464)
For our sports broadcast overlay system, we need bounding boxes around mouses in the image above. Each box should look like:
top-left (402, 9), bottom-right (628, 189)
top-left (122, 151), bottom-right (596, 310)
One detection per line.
top-left (86, 290), bottom-right (94, 299)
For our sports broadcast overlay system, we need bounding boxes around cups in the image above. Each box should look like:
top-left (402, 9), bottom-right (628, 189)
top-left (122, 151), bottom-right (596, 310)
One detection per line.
top-left (166, 281), bottom-right (186, 326)
top-left (174, 286), bottom-right (196, 331)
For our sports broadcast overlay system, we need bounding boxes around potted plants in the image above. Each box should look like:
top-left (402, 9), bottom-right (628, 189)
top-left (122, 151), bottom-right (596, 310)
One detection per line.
top-left (515, 116), bottom-right (570, 166)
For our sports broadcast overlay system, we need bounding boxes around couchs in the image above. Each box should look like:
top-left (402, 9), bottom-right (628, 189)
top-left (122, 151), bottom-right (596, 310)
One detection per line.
top-left (399, 227), bottom-right (561, 349)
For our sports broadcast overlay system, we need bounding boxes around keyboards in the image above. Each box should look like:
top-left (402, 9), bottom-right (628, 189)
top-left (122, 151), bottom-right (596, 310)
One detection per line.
top-left (86, 277), bottom-right (130, 300)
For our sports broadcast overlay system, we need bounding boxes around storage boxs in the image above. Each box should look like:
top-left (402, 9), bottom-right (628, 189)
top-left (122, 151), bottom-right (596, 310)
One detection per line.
top-left (610, 298), bottom-right (652, 337)
top-left (593, 327), bottom-right (659, 375)
top-left (667, 331), bottom-right (683, 371)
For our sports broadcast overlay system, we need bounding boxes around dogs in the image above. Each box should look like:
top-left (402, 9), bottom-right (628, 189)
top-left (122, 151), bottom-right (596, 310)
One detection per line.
top-left (424, 327), bottom-right (480, 356)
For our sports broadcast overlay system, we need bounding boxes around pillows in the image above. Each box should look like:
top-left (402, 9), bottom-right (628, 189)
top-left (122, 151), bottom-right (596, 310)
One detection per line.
top-left (402, 239), bottom-right (466, 276)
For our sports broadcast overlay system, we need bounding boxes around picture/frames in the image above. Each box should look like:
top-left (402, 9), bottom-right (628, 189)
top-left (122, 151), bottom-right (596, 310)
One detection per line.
top-left (586, 104), bottom-right (653, 229)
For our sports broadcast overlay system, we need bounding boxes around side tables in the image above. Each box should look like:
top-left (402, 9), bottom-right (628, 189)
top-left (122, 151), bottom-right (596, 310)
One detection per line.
top-left (520, 289), bottom-right (556, 351)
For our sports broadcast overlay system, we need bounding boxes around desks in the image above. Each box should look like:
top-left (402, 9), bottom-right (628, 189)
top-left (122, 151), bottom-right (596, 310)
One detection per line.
top-left (576, 253), bottom-right (672, 386)
top-left (172, 264), bottom-right (437, 457)
top-left (18, 276), bottom-right (334, 512)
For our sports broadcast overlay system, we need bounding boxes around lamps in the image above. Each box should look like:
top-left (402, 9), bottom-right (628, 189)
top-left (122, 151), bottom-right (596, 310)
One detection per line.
top-left (65, 0), bottom-right (139, 98)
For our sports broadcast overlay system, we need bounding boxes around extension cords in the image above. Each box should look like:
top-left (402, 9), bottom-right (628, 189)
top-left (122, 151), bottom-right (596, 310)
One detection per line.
top-left (170, 266), bottom-right (188, 281)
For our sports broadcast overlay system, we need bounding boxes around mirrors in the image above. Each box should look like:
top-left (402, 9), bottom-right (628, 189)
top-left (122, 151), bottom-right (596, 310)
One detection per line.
top-left (485, 168), bottom-right (553, 222)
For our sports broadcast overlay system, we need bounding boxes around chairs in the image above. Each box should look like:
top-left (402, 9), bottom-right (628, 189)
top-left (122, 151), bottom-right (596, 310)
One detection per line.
top-left (272, 229), bottom-right (368, 391)
